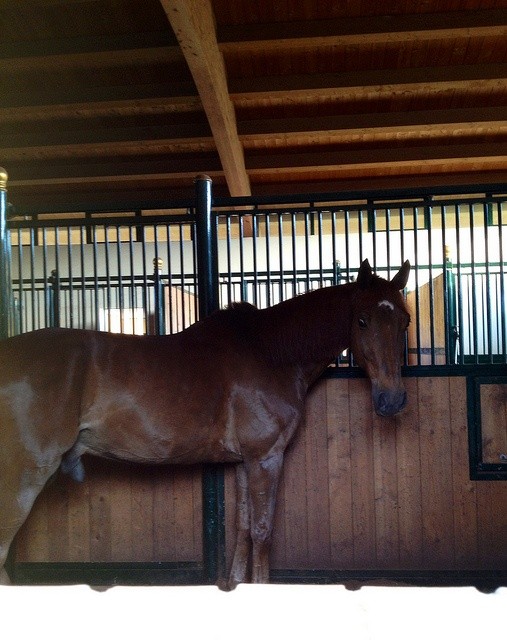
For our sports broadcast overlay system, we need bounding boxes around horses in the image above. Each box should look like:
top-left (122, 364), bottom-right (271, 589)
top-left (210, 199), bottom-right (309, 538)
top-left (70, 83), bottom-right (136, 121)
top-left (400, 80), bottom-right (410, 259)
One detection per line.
top-left (0, 257), bottom-right (413, 585)
